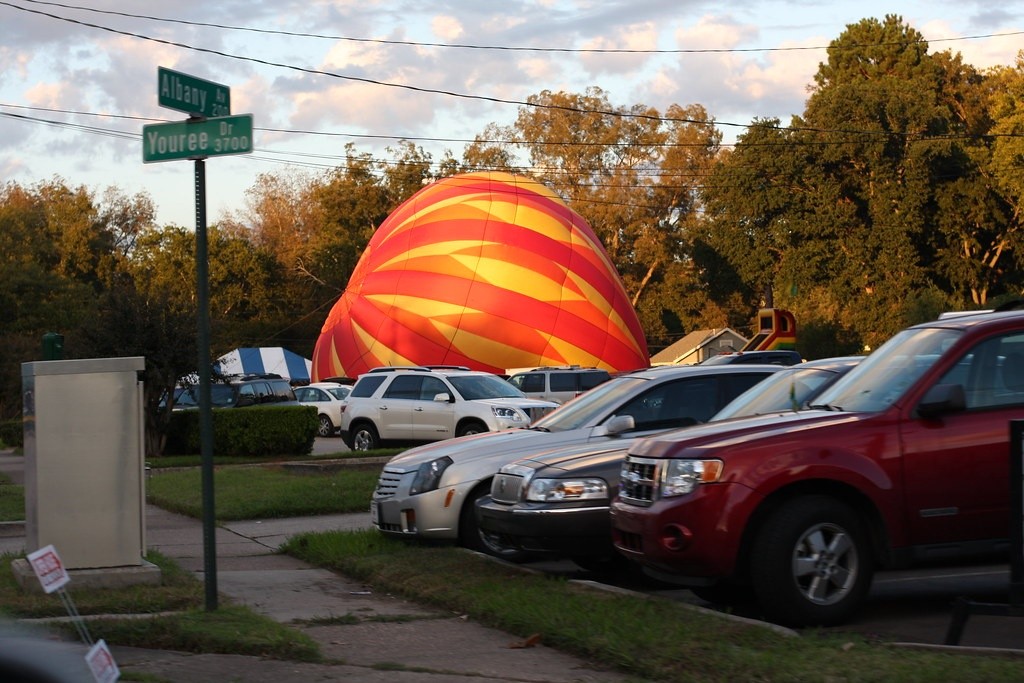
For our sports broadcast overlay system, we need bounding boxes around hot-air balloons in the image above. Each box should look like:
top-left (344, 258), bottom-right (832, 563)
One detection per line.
top-left (311, 171), bottom-right (651, 373)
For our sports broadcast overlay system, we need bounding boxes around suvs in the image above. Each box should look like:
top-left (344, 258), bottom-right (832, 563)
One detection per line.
top-left (170, 373), bottom-right (299, 430)
top-left (611, 309), bottom-right (1024, 627)
top-left (339, 364), bottom-right (560, 452)
top-left (504, 365), bottom-right (612, 408)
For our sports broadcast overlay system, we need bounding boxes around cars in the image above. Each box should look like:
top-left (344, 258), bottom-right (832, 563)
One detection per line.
top-left (370, 364), bottom-right (824, 540)
top-left (699, 349), bottom-right (803, 367)
top-left (472, 354), bottom-right (1023, 579)
top-left (290, 383), bottom-right (354, 437)
